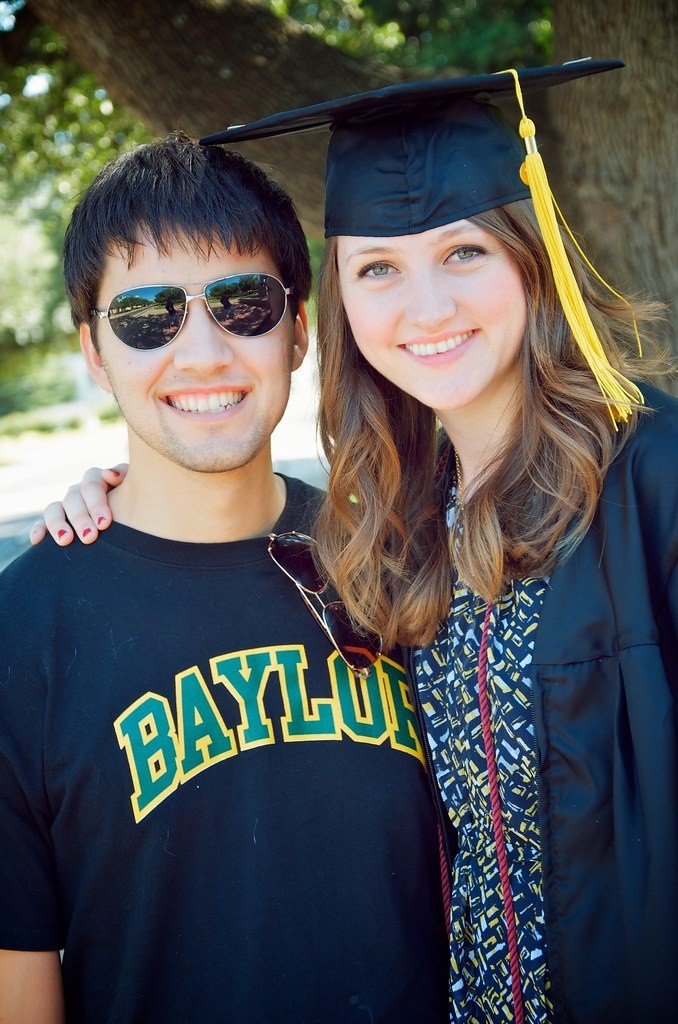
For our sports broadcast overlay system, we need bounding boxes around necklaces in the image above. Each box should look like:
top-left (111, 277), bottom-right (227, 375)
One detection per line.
top-left (451, 448), bottom-right (469, 519)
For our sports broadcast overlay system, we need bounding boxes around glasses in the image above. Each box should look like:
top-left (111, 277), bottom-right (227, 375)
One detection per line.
top-left (89, 272), bottom-right (294, 352)
top-left (268, 532), bottom-right (384, 675)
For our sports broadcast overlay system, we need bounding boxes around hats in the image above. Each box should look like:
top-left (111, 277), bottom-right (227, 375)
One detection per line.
top-left (199, 59), bottom-right (645, 432)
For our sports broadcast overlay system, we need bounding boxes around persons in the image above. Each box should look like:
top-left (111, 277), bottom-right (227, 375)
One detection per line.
top-left (29, 60), bottom-right (678, 1024)
top-left (0, 131), bottom-right (453, 1022)
